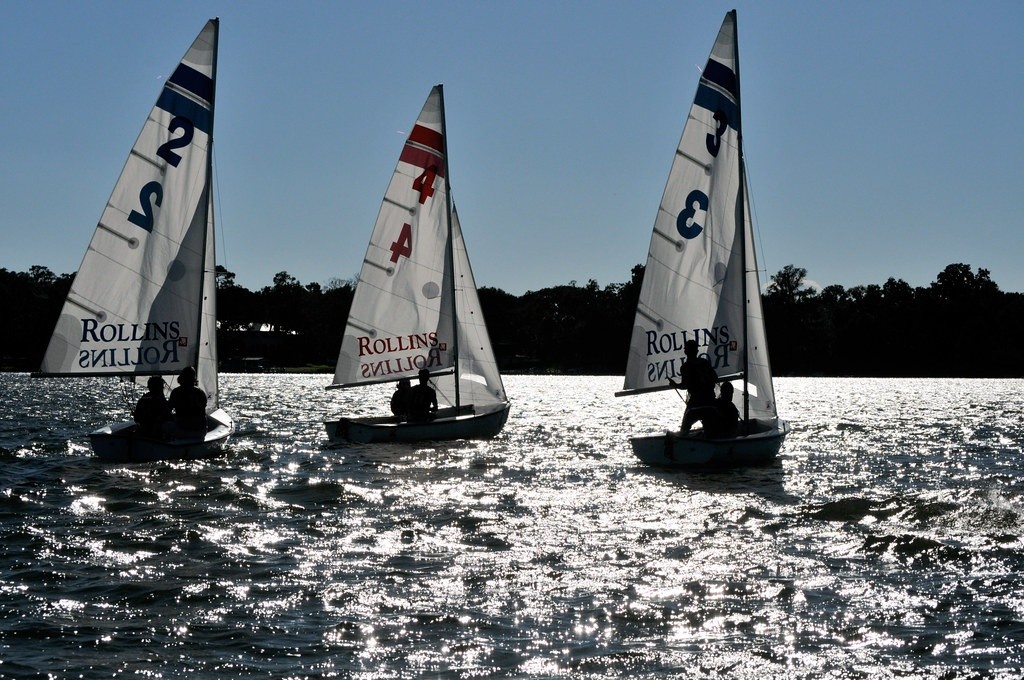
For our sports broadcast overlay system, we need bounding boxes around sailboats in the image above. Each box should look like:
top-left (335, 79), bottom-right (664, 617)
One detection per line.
top-left (27, 17), bottom-right (236, 462)
top-left (613, 9), bottom-right (793, 475)
top-left (320, 84), bottom-right (511, 444)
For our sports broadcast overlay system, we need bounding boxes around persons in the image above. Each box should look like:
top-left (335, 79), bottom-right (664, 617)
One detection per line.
top-left (391, 369), bottom-right (438, 424)
top-left (134, 366), bottom-right (207, 439)
top-left (666, 340), bottom-right (739, 431)
